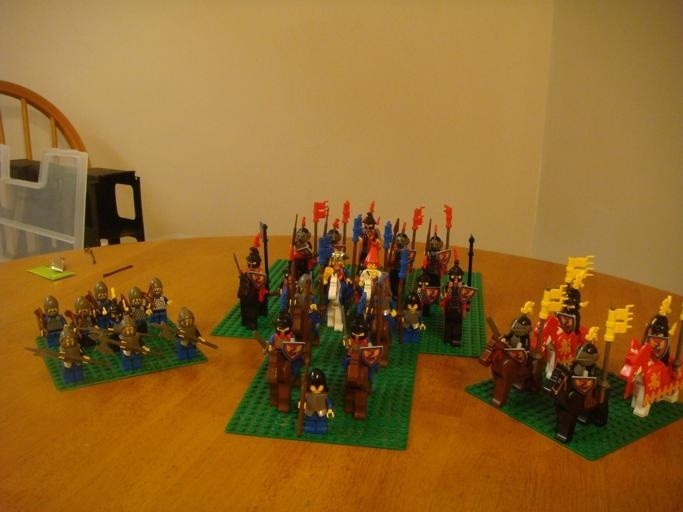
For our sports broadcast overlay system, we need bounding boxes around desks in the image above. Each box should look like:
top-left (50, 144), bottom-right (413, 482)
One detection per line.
top-left (0, 234), bottom-right (683, 512)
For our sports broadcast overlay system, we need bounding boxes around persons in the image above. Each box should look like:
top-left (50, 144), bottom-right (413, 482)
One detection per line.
top-left (30, 200), bottom-right (683, 462)
